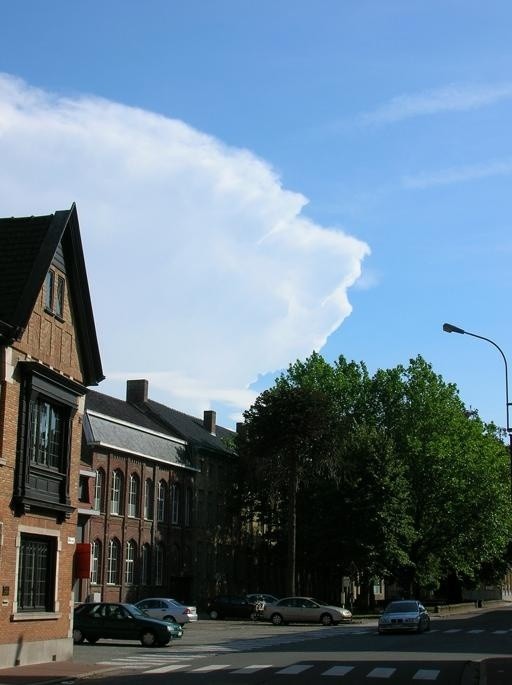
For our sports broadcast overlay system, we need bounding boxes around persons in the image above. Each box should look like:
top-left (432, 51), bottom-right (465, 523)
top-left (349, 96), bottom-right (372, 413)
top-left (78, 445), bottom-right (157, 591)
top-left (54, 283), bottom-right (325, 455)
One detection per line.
top-left (255, 597), bottom-right (266, 620)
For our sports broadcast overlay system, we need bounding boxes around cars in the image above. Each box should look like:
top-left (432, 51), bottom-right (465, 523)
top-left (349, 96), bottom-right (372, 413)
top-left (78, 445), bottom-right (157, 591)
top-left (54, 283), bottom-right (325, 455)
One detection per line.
top-left (207, 593), bottom-right (354, 625)
top-left (378, 599), bottom-right (432, 635)
top-left (74, 597), bottom-right (198, 647)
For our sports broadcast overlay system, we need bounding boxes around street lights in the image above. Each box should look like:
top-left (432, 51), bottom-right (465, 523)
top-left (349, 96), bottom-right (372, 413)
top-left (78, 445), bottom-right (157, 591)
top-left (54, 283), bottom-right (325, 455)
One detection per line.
top-left (444, 323), bottom-right (512, 454)
